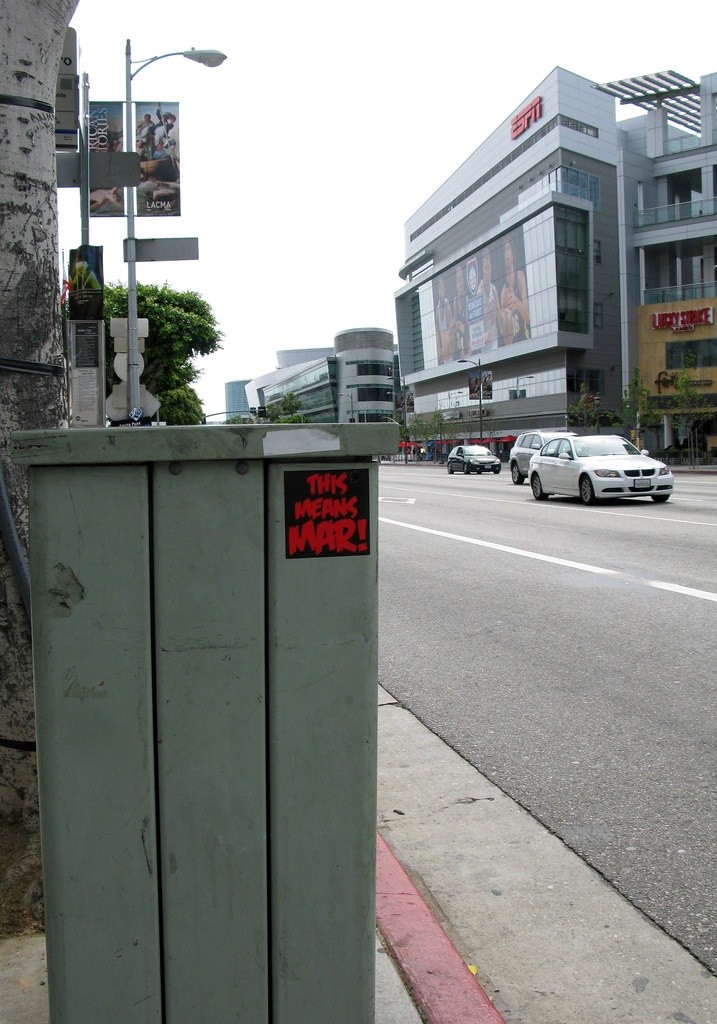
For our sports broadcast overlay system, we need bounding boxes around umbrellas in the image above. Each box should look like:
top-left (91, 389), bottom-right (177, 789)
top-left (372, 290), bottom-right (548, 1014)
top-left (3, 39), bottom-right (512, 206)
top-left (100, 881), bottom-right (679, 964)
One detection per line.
top-left (470, 439), bottom-right (481, 443)
top-left (482, 438), bottom-right (495, 444)
top-left (399, 440), bottom-right (459, 448)
top-left (496, 435), bottom-right (518, 442)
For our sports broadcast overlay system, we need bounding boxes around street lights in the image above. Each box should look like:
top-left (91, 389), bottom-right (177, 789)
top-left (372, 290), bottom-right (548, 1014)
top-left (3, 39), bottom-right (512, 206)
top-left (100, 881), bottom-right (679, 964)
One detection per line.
top-left (447, 391), bottom-right (463, 412)
top-left (592, 396), bottom-right (601, 435)
top-left (386, 392), bottom-right (404, 423)
top-left (337, 392), bottom-right (355, 424)
top-left (388, 375), bottom-right (409, 465)
top-left (516, 375), bottom-right (534, 399)
top-left (125, 40), bottom-right (229, 424)
top-left (457, 356), bottom-right (483, 447)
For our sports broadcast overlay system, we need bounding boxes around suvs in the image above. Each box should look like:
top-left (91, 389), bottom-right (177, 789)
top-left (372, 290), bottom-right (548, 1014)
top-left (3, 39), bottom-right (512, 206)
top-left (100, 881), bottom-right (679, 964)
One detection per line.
top-left (509, 429), bottom-right (592, 485)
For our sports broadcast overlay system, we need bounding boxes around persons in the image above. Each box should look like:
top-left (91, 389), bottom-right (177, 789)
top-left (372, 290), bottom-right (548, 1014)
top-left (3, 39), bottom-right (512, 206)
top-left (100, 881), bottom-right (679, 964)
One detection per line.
top-left (437, 232), bottom-right (530, 364)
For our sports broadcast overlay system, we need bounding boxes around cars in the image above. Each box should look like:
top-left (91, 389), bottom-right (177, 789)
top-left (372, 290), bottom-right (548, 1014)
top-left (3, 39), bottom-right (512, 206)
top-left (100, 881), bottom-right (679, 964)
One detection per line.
top-left (446, 444), bottom-right (502, 475)
top-left (528, 434), bottom-right (675, 506)
